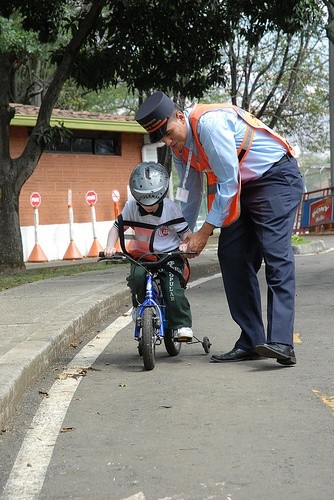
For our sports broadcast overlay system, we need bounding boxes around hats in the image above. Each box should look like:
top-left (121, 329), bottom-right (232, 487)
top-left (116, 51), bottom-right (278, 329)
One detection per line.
top-left (135, 91), bottom-right (174, 143)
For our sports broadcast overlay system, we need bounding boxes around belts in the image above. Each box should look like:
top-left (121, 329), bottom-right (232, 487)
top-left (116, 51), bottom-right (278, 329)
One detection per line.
top-left (276, 154), bottom-right (290, 163)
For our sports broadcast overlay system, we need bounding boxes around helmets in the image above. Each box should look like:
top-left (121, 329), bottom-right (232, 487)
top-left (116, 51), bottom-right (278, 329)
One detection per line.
top-left (129, 162), bottom-right (168, 206)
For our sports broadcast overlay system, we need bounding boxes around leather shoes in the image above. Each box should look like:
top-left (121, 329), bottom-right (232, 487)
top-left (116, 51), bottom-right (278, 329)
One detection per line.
top-left (210, 347), bottom-right (266, 362)
top-left (254, 342), bottom-right (296, 365)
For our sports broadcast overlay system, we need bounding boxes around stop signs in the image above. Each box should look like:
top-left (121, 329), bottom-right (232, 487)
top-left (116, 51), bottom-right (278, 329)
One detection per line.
top-left (29, 192), bottom-right (42, 209)
top-left (85, 190), bottom-right (98, 205)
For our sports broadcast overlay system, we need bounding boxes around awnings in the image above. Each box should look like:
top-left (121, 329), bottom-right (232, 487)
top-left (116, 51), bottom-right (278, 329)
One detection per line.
top-left (9, 113), bottom-right (149, 136)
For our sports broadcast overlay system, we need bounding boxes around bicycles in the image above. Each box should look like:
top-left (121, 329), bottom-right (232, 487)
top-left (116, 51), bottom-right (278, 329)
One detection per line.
top-left (96, 249), bottom-right (213, 372)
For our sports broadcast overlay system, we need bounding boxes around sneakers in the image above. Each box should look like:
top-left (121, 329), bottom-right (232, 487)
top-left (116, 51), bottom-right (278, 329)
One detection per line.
top-left (131, 308), bottom-right (138, 322)
top-left (177, 327), bottom-right (193, 338)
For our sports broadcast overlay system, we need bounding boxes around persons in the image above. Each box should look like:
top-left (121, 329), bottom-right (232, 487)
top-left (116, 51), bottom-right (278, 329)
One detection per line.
top-left (135, 90), bottom-right (303, 365)
top-left (103, 161), bottom-right (193, 341)
